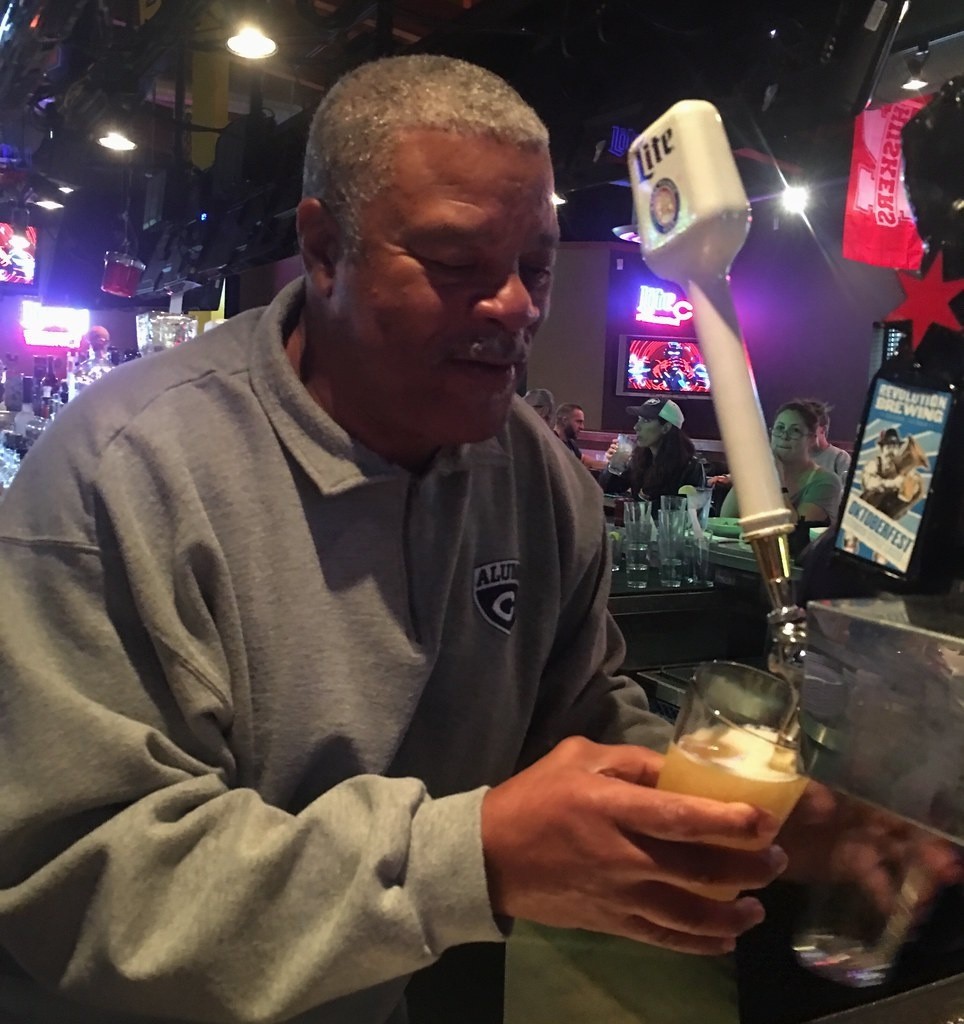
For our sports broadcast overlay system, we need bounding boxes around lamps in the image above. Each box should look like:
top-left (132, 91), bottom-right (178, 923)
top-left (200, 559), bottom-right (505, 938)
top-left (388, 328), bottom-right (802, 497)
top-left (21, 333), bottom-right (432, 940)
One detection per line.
top-left (901, 48), bottom-right (928, 94)
top-left (224, 2), bottom-right (278, 63)
top-left (95, 134), bottom-right (138, 156)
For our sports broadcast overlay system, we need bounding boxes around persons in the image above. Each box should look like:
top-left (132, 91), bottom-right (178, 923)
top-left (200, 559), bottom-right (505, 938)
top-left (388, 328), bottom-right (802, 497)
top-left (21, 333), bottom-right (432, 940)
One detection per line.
top-left (519, 388), bottom-right (852, 528)
top-left (78, 325), bottom-right (120, 393)
top-left (73, 335), bottom-right (91, 377)
top-left (860, 428), bottom-right (927, 520)
top-left (0, 52), bottom-right (955, 1024)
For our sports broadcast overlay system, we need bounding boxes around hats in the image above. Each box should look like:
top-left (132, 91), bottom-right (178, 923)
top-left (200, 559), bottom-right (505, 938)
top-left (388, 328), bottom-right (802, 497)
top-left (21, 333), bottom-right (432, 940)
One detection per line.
top-left (877, 428), bottom-right (905, 445)
top-left (626, 397), bottom-right (684, 430)
top-left (89, 326), bottom-right (110, 344)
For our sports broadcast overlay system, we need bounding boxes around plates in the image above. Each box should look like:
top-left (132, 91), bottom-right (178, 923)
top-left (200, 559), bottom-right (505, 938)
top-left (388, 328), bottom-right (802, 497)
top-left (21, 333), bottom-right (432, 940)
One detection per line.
top-left (705, 517), bottom-right (743, 535)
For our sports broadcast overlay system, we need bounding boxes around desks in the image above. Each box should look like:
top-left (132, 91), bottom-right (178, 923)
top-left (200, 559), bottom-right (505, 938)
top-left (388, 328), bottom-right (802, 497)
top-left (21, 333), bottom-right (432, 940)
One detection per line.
top-left (653, 526), bottom-right (809, 580)
top-left (608, 525), bottom-right (719, 670)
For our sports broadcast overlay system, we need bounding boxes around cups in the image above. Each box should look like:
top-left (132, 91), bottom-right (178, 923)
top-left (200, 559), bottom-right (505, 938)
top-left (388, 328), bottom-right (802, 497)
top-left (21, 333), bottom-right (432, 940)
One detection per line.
top-left (608, 434), bottom-right (636, 477)
top-left (613, 496), bottom-right (714, 590)
top-left (652, 658), bottom-right (820, 849)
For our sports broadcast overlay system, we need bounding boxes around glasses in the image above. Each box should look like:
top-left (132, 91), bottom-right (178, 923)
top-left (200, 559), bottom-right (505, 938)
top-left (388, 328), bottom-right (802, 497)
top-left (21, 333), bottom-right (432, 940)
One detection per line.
top-left (769, 427), bottom-right (813, 440)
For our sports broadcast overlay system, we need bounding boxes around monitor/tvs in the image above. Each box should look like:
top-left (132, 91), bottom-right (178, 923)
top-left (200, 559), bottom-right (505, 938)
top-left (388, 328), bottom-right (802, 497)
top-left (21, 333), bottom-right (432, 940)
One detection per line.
top-left (615, 335), bottom-right (711, 400)
top-left (0, 218), bottom-right (43, 296)
